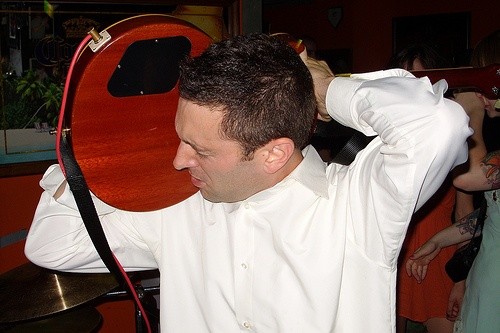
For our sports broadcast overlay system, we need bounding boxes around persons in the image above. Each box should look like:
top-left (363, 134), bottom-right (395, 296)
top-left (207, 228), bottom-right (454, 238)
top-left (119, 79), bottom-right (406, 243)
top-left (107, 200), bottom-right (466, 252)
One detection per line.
top-left (396, 36), bottom-right (500, 333)
top-left (24, 32), bottom-right (475, 333)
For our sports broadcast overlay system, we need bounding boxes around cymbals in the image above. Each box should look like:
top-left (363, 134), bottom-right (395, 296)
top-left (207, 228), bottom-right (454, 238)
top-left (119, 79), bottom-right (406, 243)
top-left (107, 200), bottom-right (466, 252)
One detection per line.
top-left (0, 261), bottom-right (135, 322)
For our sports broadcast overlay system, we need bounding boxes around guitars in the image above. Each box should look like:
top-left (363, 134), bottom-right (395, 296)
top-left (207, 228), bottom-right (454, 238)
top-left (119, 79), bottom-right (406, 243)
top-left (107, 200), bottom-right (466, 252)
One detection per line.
top-left (48, 12), bottom-right (500, 212)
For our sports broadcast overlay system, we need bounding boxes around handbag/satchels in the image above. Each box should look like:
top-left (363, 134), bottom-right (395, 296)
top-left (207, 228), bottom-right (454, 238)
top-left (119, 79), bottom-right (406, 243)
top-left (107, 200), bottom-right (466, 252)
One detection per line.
top-left (445, 239), bottom-right (486, 283)
